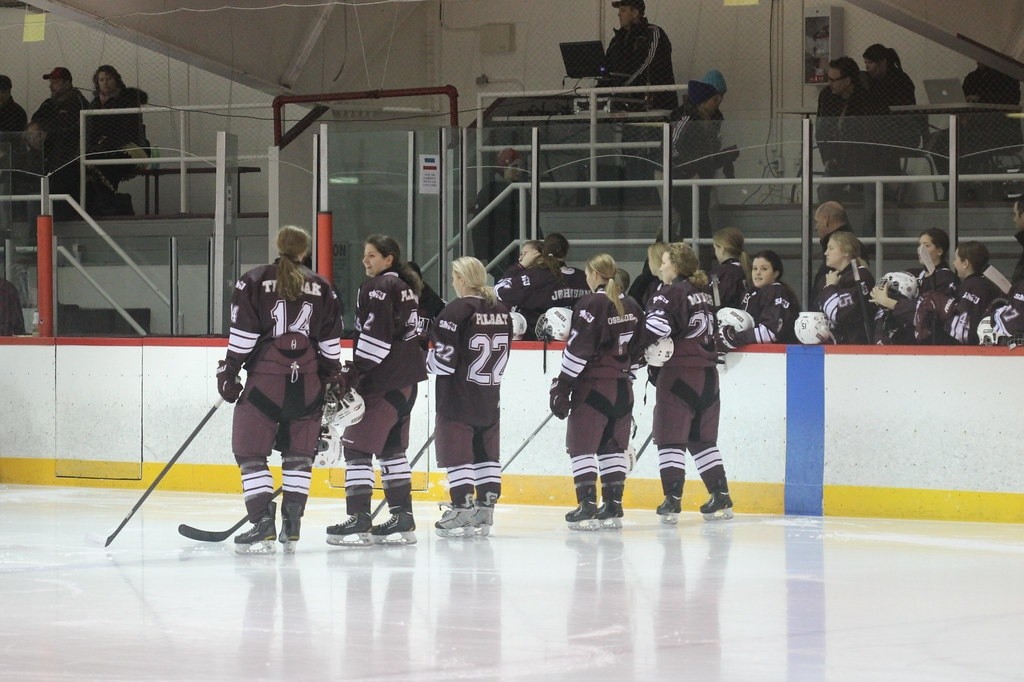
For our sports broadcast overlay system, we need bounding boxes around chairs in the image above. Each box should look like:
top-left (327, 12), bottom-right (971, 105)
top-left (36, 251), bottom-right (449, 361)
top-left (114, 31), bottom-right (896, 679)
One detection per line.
top-left (814, 94), bottom-right (1024, 207)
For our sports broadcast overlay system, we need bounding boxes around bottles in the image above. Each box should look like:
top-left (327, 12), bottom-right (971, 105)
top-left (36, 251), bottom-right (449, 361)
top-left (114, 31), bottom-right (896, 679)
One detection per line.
top-left (32, 311), bottom-right (39, 336)
top-left (151, 144), bottom-right (160, 170)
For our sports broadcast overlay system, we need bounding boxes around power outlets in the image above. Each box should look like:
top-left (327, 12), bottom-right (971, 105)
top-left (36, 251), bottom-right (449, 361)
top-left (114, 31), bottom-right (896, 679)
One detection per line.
top-left (774, 158), bottom-right (785, 172)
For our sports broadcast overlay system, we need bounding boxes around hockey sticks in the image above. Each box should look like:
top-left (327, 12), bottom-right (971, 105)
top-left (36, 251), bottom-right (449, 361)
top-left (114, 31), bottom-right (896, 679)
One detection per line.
top-left (439, 412), bottom-right (554, 512)
top-left (712, 272), bottom-right (721, 311)
top-left (177, 487), bottom-right (283, 542)
top-left (90, 375), bottom-right (242, 547)
top-left (979, 263), bottom-right (1013, 298)
top-left (636, 430), bottom-right (653, 462)
top-left (371, 430), bottom-right (437, 520)
top-left (918, 244), bottom-right (939, 345)
top-left (850, 257), bottom-right (872, 344)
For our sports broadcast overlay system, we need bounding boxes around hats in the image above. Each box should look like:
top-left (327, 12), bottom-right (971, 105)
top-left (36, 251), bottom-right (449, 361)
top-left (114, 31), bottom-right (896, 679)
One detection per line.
top-left (704, 70), bottom-right (727, 93)
top-left (43, 67), bottom-right (72, 80)
top-left (497, 148), bottom-right (522, 172)
top-left (612, 0), bottom-right (645, 8)
top-left (687, 79), bottom-right (719, 103)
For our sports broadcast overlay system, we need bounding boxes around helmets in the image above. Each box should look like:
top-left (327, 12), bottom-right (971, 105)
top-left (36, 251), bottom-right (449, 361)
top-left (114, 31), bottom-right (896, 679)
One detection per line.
top-left (794, 311), bottom-right (830, 344)
top-left (325, 387), bottom-right (366, 426)
top-left (312, 424), bottom-right (341, 467)
top-left (644, 337), bottom-right (673, 366)
top-left (716, 307), bottom-right (755, 350)
top-left (881, 271), bottom-right (918, 299)
top-left (510, 312), bottom-right (527, 340)
top-left (976, 316), bottom-right (1006, 345)
top-left (543, 307), bottom-right (574, 341)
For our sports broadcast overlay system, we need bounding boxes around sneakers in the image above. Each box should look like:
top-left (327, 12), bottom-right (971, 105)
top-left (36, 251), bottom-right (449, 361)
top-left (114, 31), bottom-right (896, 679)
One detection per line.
top-left (371, 513), bottom-right (418, 544)
top-left (234, 502), bottom-right (277, 553)
top-left (564, 487), bottom-right (600, 530)
top-left (325, 512), bottom-right (375, 547)
top-left (434, 493), bottom-right (476, 537)
top-left (472, 492), bottom-right (498, 536)
top-left (656, 492), bottom-right (682, 525)
top-left (278, 504), bottom-right (300, 553)
top-left (596, 486), bottom-right (625, 528)
top-left (699, 491), bottom-right (735, 521)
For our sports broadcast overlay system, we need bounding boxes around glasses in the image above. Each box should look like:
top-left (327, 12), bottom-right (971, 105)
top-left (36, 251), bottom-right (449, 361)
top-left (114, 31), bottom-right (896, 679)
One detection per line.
top-left (827, 75), bottom-right (847, 83)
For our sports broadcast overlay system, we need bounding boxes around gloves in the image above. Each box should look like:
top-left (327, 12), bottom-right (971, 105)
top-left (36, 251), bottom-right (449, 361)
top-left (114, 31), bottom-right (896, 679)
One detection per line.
top-left (340, 360), bottom-right (365, 394)
top-left (647, 365), bottom-right (660, 386)
top-left (216, 360), bottom-right (243, 404)
top-left (549, 378), bottom-right (572, 419)
top-left (320, 355), bottom-right (343, 383)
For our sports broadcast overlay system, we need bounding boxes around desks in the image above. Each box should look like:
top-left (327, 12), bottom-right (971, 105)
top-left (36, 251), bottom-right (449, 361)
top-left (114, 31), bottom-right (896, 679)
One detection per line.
top-left (467, 96), bottom-right (648, 206)
top-left (775, 103), bottom-right (1020, 205)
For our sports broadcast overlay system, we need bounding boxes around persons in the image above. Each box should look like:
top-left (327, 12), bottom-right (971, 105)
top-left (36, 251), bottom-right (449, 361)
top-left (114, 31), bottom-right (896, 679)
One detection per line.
top-left (426, 256), bottom-right (513, 536)
top-left (640, 244), bottom-right (735, 525)
top-left (215, 226), bottom-right (341, 542)
top-left (326, 234), bottom-right (425, 544)
top-left (548, 254), bottom-right (638, 532)
top-left (0, 64), bottom-right (151, 339)
top-left (470, 0), bottom-right (1023, 346)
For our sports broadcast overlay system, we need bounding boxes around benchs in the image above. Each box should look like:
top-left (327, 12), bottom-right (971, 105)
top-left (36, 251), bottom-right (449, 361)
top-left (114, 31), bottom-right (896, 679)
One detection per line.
top-left (136, 166), bottom-right (260, 214)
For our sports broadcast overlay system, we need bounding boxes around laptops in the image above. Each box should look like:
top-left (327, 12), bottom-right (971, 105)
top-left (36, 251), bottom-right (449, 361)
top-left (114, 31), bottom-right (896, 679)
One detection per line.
top-left (923, 78), bottom-right (967, 104)
top-left (559, 41), bottom-right (632, 78)
